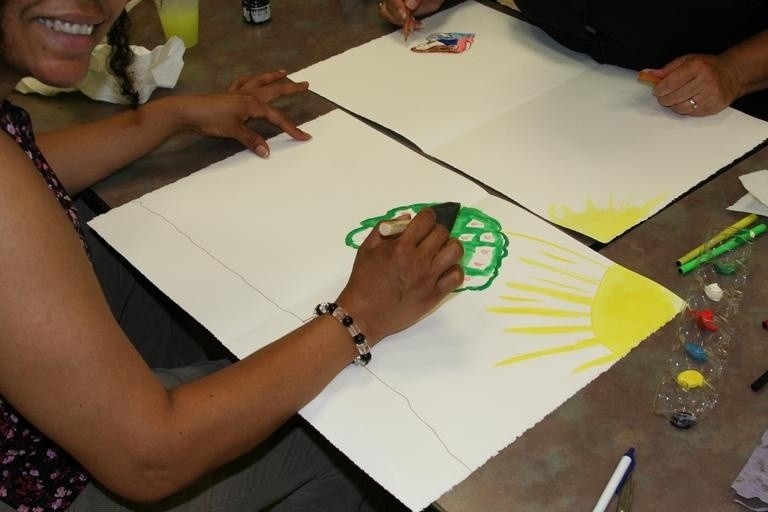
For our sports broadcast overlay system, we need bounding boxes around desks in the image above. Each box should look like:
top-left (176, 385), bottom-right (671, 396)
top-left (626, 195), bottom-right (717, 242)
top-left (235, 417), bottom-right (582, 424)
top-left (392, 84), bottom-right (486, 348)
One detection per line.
top-left (0, 0), bottom-right (768, 512)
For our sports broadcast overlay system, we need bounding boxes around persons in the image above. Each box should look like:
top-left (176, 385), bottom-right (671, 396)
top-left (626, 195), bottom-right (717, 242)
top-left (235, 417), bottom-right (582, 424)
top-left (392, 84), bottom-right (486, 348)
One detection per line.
top-left (1, 0), bottom-right (465, 511)
top-left (378, 1), bottom-right (768, 117)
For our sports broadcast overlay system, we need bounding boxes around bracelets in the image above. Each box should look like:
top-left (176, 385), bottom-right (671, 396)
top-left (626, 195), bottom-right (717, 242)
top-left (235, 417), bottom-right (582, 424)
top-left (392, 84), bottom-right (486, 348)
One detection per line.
top-left (312, 303), bottom-right (373, 366)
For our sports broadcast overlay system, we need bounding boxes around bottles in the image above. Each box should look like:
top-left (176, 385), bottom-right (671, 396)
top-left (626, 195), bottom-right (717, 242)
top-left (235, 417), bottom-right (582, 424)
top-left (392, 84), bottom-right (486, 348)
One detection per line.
top-left (243, 0), bottom-right (271, 24)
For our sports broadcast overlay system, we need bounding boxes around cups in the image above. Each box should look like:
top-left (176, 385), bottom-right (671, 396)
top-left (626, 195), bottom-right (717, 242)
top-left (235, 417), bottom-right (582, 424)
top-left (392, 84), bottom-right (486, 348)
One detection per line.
top-left (151, 1), bottom-right (200, 51)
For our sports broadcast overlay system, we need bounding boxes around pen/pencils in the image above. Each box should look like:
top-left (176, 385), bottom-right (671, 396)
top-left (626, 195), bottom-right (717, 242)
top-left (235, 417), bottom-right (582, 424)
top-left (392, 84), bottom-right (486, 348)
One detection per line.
top-left (592, 447), bottom-right (637, 512)
top-left (751, 370), bottom-right (768, 392)
top-left (762, 319), bottom-right (768, 328)
top-left (637, 71), bottom-right (663, 85)
top-left (404, 0), bottom-right (410, 41)
top-left (676, 214), bottom-right (768, 274)
top-left (379, 219), bottom-right (412, 237)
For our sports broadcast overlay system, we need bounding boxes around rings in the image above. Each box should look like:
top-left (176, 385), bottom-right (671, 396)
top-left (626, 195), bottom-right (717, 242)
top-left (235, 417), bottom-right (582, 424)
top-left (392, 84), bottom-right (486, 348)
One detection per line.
top-left (690, 99), bottom-right (699, 110)
